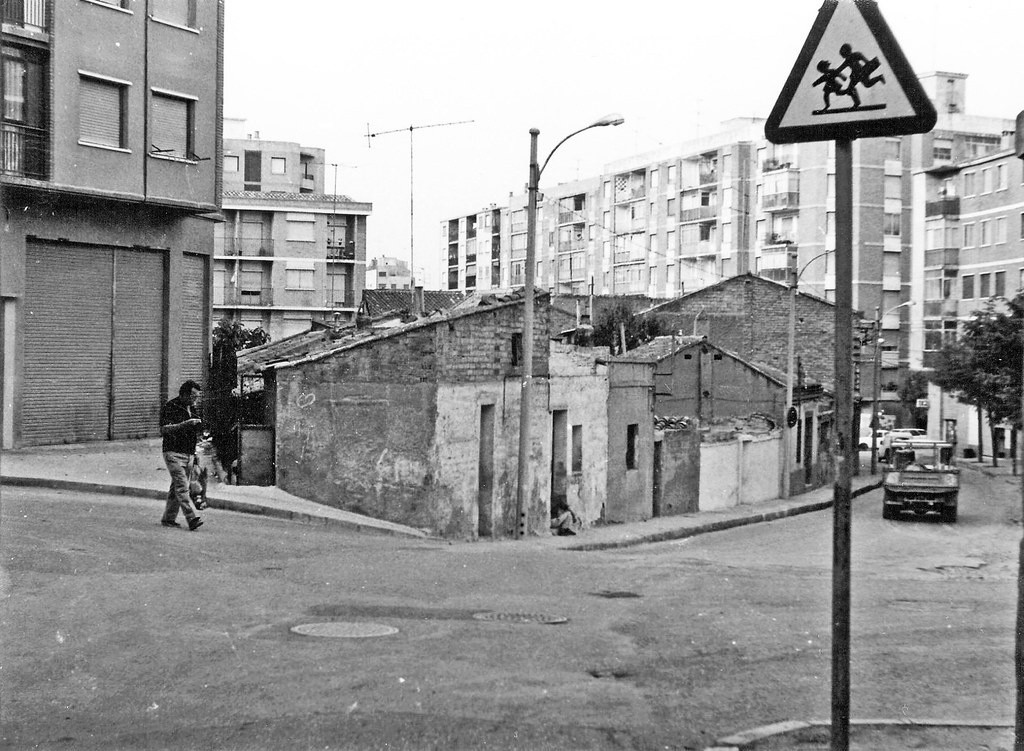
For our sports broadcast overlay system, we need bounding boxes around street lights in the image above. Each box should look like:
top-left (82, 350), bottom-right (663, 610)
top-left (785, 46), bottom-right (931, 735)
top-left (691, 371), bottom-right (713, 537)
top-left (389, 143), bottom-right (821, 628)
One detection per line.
top-left (516, 108), bottom-right (627, 540)
top-left (870, 301), bottom-right (916, 476)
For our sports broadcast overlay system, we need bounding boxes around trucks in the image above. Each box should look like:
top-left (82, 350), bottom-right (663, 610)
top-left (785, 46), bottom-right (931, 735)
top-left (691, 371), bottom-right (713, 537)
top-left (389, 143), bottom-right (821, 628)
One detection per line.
top-left (859, 412), bottom-right (896, 451)
top-left (882, 437), bottom-right (960, 524)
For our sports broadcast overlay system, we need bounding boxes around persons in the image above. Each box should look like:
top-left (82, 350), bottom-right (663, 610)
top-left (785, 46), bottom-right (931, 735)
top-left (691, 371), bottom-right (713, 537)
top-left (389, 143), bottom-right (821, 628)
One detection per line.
top-left (159, 380), bottom-right (203, 531)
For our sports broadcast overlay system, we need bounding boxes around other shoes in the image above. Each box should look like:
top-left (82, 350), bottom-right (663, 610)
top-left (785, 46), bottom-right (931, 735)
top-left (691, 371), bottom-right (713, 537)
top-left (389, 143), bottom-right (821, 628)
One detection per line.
top-left (161, 520), bottom-right (180, 527)
top-left (559, 527), bottom-right (576, 536)
top-left (189, 519), bottom-right (203, 531)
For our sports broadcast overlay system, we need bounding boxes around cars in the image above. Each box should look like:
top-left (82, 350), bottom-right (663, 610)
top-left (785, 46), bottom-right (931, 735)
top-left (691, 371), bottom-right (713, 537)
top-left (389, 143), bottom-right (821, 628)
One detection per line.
top-left (876, 427), bottom-right (928, 464)
